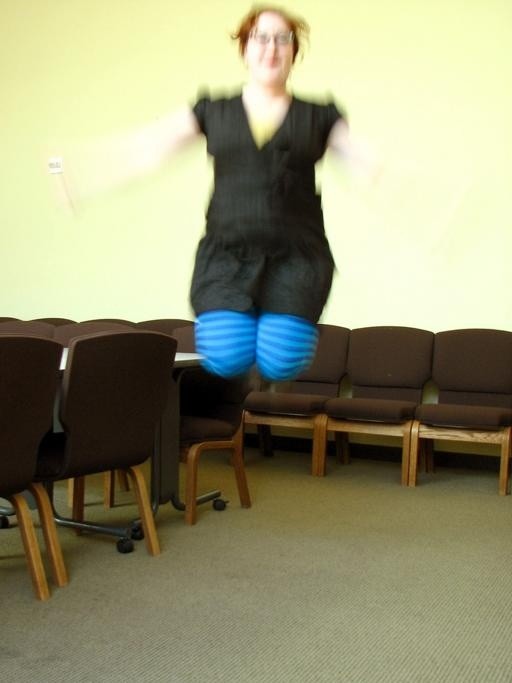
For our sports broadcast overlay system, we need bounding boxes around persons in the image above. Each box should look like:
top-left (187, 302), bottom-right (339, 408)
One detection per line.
top-left (57, 3), bottom-right (373, 380)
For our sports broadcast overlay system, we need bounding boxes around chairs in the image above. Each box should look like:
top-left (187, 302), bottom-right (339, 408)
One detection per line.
top-left (244, 324), bottom-right (350, 477)
top-left (319, 324), bottom-right (435, 488)
top-left (1, 315), bottom-right (262, 599)
top-left (410, 326), bottom-right (512, 498)
